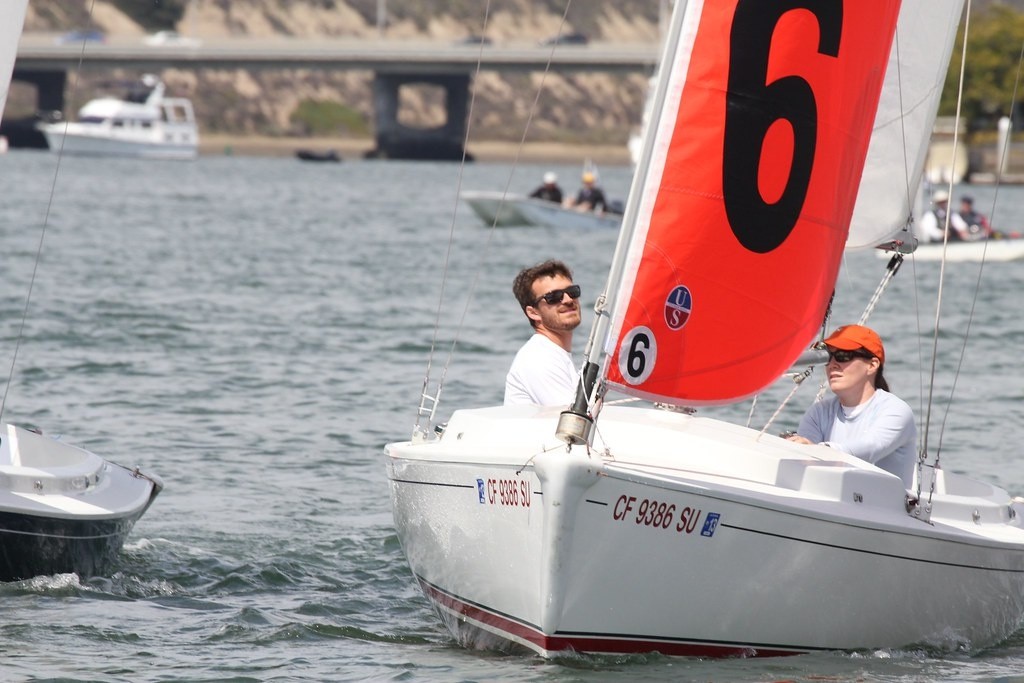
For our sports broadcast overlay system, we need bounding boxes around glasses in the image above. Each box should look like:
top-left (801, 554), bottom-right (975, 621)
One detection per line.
top-left (810, 341), bottom-right (872, 366)
top-left (530, 285), bottom-right (581, 306)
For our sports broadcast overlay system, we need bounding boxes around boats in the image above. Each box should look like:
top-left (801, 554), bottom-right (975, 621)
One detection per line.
top-left (513, 198), bottom-right (618, 238)
top-left (454, 189), bottom-right (534, 226)
top-left (38, 77), bottom-right (199, 166)
top-left (872, 231), bottom-right (1023, 263)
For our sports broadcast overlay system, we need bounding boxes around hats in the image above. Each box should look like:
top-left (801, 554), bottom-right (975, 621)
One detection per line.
top-left (582, 172), bottom-right (594, 183)
top-left (933, 191), bottom-right (948, 202)
top-left (823, 324), bottom-right (884, 366)
top-left (543, 172), bottom-right (557, 184)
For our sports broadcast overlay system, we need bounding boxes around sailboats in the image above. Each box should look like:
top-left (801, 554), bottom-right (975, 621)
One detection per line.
top-left (1, 1), bottom-right (162, 577)
top-left (381, 1), bottom-right (1024, 656)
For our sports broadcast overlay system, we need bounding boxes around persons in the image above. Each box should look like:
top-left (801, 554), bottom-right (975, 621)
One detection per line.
top-left (787, 324), bottom-right (917, 489)
top-left (960, 195), bottom-right (993, 235)
top-left (504, 261), bottom-right (582, 408)
top-left (922, 192), bottom-right (969, 242)
top-left (531, 173), bottom-right (563, 202)
top-left (565, 172), bottom-right (608, 218)
